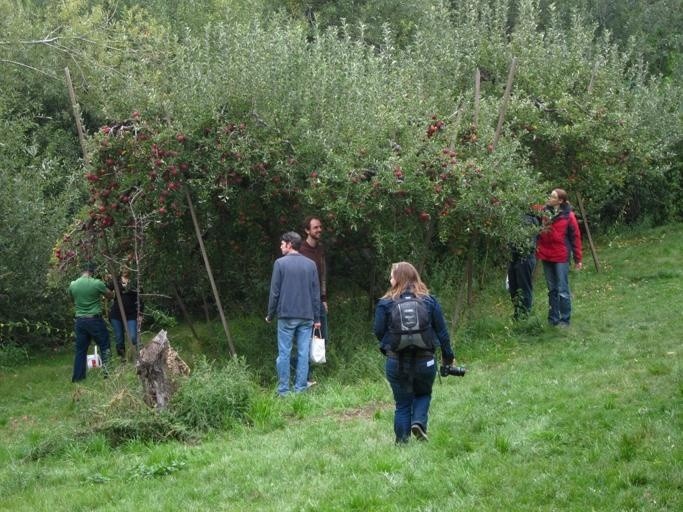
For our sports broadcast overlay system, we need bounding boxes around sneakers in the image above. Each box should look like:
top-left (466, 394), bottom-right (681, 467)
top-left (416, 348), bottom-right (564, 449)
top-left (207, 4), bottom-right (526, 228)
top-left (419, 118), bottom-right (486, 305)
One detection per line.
top-left (410, 423), bottom-right (429, 443)
top-left (306, 381), bottom-right (316, 388)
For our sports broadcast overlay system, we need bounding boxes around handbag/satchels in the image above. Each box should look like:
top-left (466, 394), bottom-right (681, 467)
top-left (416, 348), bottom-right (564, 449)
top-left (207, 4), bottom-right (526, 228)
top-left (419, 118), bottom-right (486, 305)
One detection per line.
top-left (86, 344), bottom-right (102, 369)
top-left (309, 327), bottom-right (326, 365)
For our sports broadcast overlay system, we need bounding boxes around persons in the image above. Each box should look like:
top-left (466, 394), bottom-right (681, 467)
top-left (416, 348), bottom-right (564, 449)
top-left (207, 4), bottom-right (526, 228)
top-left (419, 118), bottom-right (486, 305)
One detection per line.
top-left (291, 216), bottom-right (329, 371)
top-left (104, 260), bottom-right (144, 362)
top-left (66, 263), bottom-right (114, 385)
top-left (372, 262), bottom-right (456, 446)
top-left (506, 209), bottom-right (541, 321)
top-left (530, 188), bottom-right (584, 331)
top-left (265, 232), bottom-right (321, 400)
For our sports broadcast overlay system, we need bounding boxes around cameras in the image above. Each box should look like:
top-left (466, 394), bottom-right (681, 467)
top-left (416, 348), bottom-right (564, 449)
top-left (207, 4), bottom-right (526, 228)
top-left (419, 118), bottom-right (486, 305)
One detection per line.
top-left (440, 363), bottom-right (466, 378)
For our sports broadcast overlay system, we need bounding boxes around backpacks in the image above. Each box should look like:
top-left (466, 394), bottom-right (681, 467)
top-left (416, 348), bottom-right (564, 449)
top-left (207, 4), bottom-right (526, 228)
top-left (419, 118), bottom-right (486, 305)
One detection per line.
top-left (375, 294), bottom-right (435, 394)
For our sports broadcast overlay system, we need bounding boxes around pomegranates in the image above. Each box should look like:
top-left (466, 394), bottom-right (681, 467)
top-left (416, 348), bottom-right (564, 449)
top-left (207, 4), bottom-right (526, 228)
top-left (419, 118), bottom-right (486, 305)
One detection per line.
top-left (56, 109), bottom-right (560, 272)
